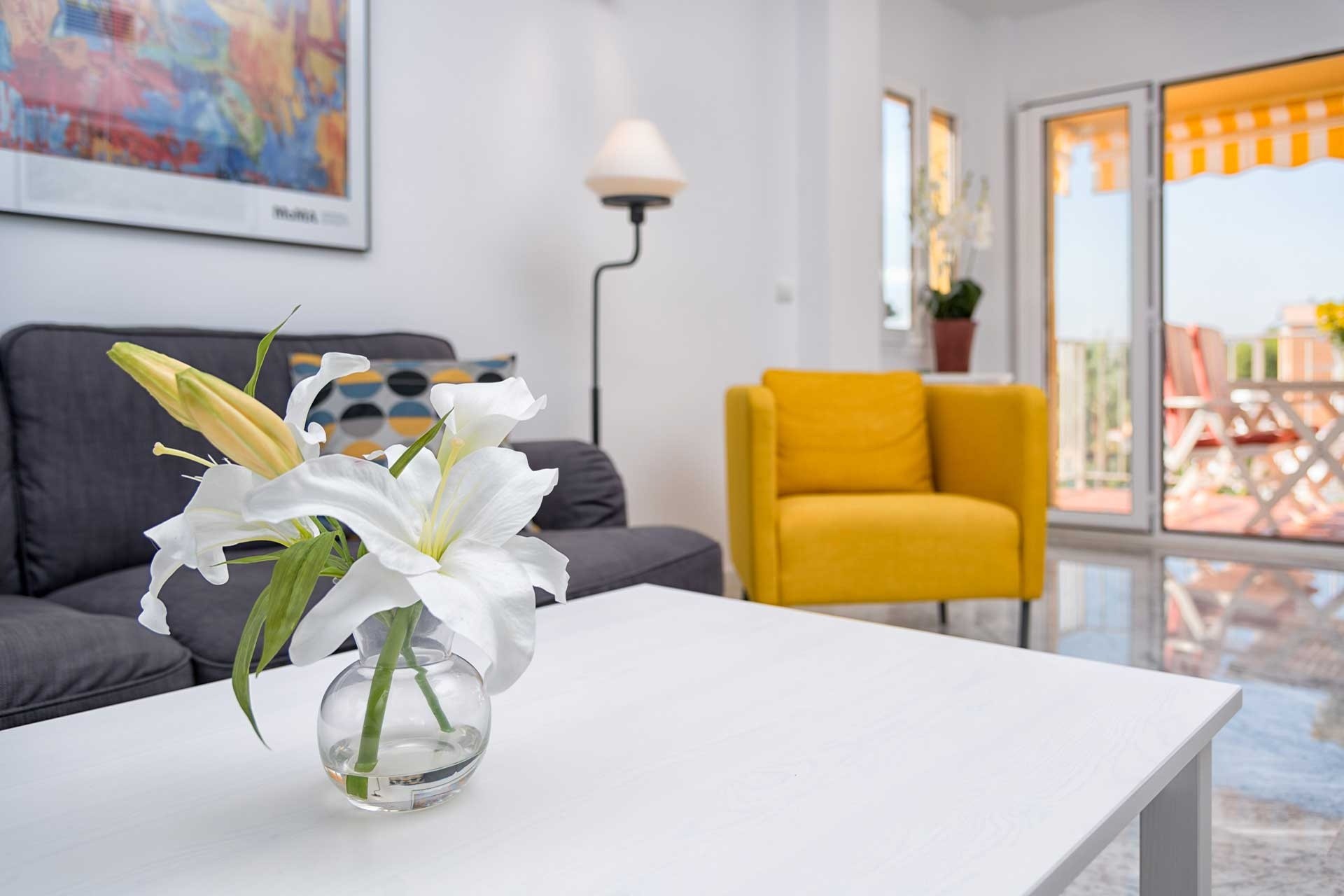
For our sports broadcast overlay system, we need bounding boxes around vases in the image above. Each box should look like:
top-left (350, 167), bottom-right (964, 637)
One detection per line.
top-left (930, 317), bottom-right (975, 373)
top-left (317, 610), bottom-right (491, 814)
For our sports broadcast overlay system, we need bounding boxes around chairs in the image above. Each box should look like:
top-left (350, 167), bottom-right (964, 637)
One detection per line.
top-left (1157, 321), bottom-right (1323, 537)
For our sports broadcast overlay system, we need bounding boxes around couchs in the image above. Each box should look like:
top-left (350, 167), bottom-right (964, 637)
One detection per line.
top-left (3, 323), bottom-right (728, 733)
top-left (725, 368), bottom-right (1051, 649)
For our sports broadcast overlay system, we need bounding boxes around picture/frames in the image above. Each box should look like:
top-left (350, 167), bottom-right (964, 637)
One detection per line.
top-left (1, 0), bottom-right (371, 252)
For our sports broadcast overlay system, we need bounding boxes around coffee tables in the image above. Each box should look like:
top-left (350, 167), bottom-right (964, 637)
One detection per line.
top-left (1, 581), bottom-right (1244, 896)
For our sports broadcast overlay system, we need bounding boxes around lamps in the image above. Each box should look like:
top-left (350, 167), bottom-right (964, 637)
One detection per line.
top-left (586, 119), bottom-right (692, 445)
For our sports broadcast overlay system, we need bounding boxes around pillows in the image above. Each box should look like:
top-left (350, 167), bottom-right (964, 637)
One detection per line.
top-left (286, 351), bottom-right (517, 536)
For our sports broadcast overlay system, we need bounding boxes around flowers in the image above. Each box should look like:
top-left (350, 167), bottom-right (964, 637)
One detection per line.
top-left (914, 167), bottom-right (986, 322)
top-left (1316, 301), bottom-right (1344, 356)
top-left (103, 303), bottom-right (572, 798)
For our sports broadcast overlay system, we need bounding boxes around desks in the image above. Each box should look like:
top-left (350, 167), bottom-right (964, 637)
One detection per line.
top-left (1226, 379), bottom-right (1344, 531)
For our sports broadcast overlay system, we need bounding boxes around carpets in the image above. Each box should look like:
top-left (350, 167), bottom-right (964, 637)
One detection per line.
top-left (1064, 778), bottom-right (1343, 896)
